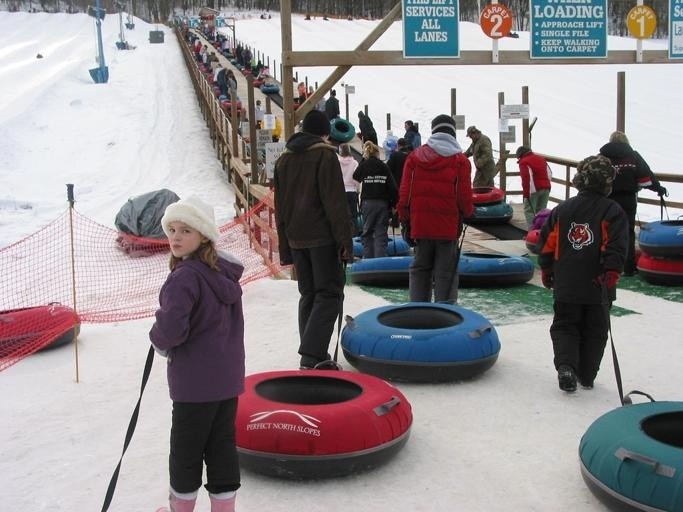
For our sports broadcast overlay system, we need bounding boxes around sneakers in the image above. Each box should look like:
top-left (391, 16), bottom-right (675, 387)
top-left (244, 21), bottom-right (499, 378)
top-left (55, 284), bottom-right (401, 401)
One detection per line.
top-left (557, 365), bottom-right (593, 391)
top-left (621, 268), bottom-right (638, 277)
top-left (300, 352), bottom-right (343, 370)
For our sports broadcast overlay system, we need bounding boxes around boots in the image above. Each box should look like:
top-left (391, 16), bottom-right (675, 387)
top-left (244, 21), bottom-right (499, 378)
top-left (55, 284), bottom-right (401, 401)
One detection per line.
top-left (156, 486), bottom-right (237, 512)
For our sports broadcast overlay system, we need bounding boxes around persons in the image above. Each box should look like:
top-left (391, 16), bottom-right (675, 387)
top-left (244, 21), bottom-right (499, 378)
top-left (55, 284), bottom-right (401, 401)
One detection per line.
top-left (182, 7), bottom-right (378, 146)
top-left (386, 120), bottom-right (421, 227)
top-left (463, 125), bottom-right (495, 192)
top-left (535, 154), bottom-right (629, 392)
top-left (27, 5), bottom-right (80, 14)
top-left (148, 192), bottom-right (245, 512)
top-left (597, 131), bottom-right (666, 277)
top-left (353, 140), bottom-right (398, 258)
top-left (273, 109), bottom-right (354, 371)
top-left (397, 114), bottom-right (476, 304)
top-left (515, 146), bottom-right (553, 232)
top-left (335, 143), bottom-right (363, 238)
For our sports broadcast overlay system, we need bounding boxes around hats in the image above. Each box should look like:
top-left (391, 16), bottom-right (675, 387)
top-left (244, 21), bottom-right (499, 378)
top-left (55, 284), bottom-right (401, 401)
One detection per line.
top-left (574, 155), bottom-right (617, 198)
top-left (610, 131), bottom-right (628, 144)
top-left (466, 126), bottom-right (476, 137)
top-left (161, 194), bottom-right (223, 244)
top-left (431, 114), bottom-right (456, 139)
top-left (516, 146), bottom-right (532, 159)
top-left (303, 110), bottom-right (331, 136)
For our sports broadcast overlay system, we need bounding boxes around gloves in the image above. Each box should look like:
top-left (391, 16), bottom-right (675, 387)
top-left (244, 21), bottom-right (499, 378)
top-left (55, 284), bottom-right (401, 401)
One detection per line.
top-left (592, 271), bottom-right (619, 289)
top-left (657, 185), bottom-right (668, 196)
top-left (400, 220), bottom-right (418, 247)
top-left (542, 267), bottom-right (556, 289)
